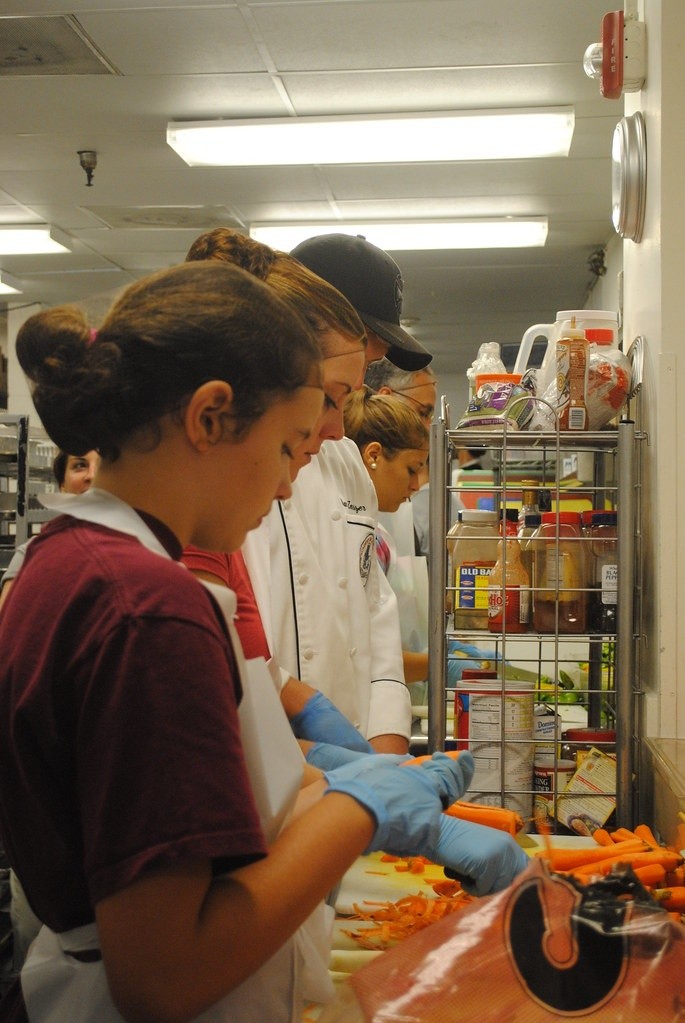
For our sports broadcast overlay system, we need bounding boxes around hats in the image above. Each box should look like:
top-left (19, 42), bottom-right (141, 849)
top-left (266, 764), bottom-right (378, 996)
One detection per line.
top-left (289, 233), bottom-right (432, 371)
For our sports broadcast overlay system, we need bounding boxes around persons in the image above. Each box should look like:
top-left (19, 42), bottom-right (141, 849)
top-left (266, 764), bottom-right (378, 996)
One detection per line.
top-left (130, 225), bottom-right (529, 896)
top-left (0, 258), bottom-right (475, 1023)
top-left (0, 450), bottom-right (102, 619)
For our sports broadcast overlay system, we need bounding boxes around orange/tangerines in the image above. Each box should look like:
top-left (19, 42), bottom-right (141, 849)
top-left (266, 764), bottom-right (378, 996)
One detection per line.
top-left (535, 692), bottom-right (608, 724)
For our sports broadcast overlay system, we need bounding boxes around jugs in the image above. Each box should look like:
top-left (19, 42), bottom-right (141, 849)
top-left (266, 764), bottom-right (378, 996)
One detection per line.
top-left (512, 309), bottom-right (620, 396)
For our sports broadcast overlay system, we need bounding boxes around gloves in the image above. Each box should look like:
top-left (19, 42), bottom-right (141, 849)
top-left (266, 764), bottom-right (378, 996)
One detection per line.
top-left (418, 751), bottom-right (474, 810)
top-left (293, 692), bottom-right (373, 753)
top-left (308, 743), bottom-right (414, 771)
top-left (324, 767), bottom-right (442, 854)
top-left (427, 812), bottom-right (532, 896)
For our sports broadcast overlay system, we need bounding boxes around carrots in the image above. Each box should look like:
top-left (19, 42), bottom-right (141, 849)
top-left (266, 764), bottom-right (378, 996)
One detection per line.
top-left (400, 750), bottom-right (463, 765)
top-left (381, 801), bottom-right (685, 929)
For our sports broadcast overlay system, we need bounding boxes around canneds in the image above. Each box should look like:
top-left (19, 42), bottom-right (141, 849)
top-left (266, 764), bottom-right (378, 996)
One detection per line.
top-left (535, 729), bottom-right (617, 829)
top-left (446, 506), bottom-right (616, 634)
top-left (454, 670), bottom-right (535, 833)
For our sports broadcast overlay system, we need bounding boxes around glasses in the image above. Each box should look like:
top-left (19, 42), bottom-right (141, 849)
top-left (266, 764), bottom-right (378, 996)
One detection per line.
top-left (386, 384), bottom-right (434, 425)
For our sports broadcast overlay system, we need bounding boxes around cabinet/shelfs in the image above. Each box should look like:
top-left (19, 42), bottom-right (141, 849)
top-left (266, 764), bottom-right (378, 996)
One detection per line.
top-left (426, 406), bottom-right (639, 836)
top-left (0, 412), bottom-right (61, 579)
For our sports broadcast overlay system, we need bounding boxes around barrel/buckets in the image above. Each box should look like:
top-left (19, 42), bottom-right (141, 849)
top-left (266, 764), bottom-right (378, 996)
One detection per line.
top-left (455, 679), bottom-right (576, 836)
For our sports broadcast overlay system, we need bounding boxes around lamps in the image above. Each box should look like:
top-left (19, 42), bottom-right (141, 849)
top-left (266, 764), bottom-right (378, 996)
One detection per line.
top-left (165, 105), bottom-right (576, 167)
top-left (0, 223), bottom-right (73, 254)
top-left (249, 215), bottom-right (548, 252)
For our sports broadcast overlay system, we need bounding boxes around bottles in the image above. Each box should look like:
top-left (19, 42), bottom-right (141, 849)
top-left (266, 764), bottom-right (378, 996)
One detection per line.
top-left (562, 725), bottom-right (618, 766)
top-left (447, 478), bottom-right (617, 642)
top-left (555, 315), bottom-right (632, 431)
